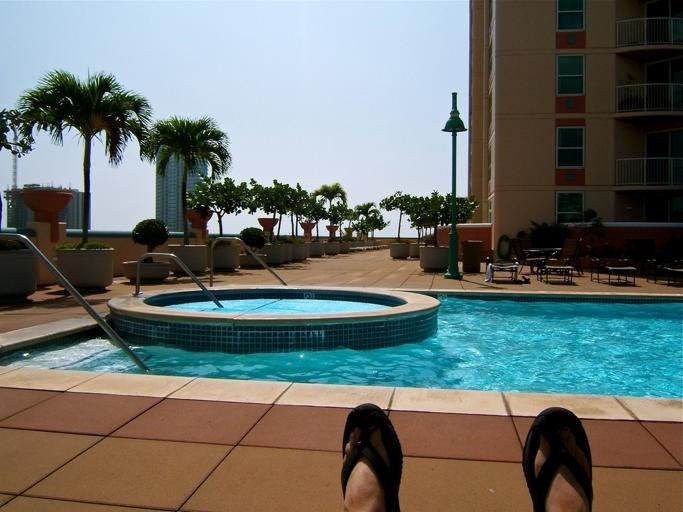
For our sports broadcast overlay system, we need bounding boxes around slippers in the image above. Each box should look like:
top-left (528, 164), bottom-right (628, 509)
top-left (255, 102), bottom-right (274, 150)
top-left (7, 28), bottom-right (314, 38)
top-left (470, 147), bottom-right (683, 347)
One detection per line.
top-left (340, 403), bottom-right (402, 511)
top-left (522, 406), bottom-right (592, 511)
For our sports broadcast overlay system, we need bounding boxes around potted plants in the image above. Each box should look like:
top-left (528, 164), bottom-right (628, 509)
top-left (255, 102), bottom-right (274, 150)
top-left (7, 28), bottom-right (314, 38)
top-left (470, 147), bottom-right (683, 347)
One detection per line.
top-left (51, 240), bottom-right (117, 296)
top-left (419, 234), bottom-right (450, 273)
top-left (239, 225), bottom-right (272, 271)
top-left (268, 234), bottom-right (353, 265)
top-left (168, 238), bottom-right (213, 279)
top-left (389, 237), bottom-right (410, 258)
top-left (118, 218), bottom-right (179, 284)
top-left (204, 235), bottom-right (247, 274)
top-left (590, 217), bottom-right (605, 233)
top-left (0, 227), bottom-right (43, 305)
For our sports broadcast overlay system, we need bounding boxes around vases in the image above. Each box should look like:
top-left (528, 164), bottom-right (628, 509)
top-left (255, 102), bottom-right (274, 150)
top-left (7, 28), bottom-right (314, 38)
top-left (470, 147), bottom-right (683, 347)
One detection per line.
top-left (409, 242), bottom-right (425, 258)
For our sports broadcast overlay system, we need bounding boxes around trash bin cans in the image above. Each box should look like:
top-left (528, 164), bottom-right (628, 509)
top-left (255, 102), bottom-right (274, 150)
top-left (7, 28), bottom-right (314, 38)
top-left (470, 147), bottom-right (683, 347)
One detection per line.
top-left (2, 227), bottom-right (37, 306)
top-left (462, 241), bottom-right (483, 273)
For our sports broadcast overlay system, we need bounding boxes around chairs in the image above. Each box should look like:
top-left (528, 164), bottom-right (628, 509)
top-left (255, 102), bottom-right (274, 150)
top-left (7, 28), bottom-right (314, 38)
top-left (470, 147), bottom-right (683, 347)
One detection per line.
top-left (486, 234), bottom-right (683, 288)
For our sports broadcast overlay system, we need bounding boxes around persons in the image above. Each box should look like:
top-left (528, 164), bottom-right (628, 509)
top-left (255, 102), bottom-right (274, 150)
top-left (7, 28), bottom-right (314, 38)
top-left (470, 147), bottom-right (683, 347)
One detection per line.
top-left (338, 401), bottom-right (594, 512)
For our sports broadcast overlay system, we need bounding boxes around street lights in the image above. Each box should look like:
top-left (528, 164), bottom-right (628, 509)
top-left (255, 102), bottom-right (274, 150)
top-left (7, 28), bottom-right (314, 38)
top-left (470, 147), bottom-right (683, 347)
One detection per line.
top-left (441, 91), bottom-right (467, 281)
